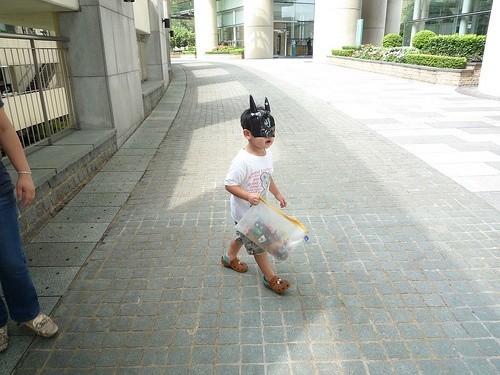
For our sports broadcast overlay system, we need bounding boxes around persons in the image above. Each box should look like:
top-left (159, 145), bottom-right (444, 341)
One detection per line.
top-left (0, 96), bottom-right (58, 350)
top-left (220, 107), bottom-right (292, 293)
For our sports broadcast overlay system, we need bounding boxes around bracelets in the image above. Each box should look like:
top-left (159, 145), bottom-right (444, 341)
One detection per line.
top-left (17, 171), bottom-right (32, 174)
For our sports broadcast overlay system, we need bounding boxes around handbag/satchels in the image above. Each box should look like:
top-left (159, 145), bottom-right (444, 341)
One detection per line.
top-left (234, 195), bottom-right (309, 262)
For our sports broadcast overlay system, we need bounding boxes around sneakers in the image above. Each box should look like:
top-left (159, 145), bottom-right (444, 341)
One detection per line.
top-left (21, 311), bottom-right (59, 338)
top-left (0, 320), bottom-right (9, 353)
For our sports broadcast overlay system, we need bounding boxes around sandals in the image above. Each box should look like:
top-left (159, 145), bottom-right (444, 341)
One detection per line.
top-left (263, 274), bottom-right (291, 294)
top-left (221, 252), bottom-right (249, 273)
